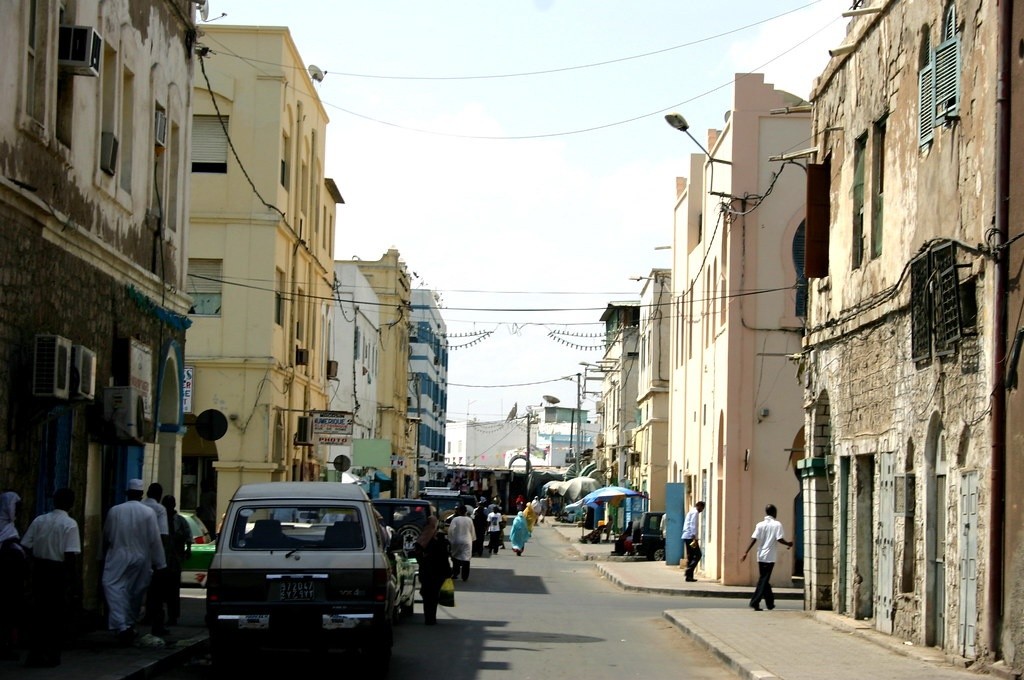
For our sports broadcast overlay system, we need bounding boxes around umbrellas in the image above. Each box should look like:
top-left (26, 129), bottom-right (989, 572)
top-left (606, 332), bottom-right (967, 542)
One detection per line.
top-left (583, 485), bottom-right (647, 506)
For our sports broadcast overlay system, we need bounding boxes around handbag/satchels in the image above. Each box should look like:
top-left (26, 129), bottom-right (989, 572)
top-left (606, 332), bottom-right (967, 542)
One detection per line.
top-left (438, 577), bottom-right (455, 607)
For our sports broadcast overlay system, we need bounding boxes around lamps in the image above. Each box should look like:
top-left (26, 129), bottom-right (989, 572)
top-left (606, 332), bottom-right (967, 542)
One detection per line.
top-left (666, 114), bottom-right (713, 162)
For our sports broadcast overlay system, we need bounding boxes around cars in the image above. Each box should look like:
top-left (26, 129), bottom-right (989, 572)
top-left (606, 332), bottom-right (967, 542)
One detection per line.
top-left (175, 508), bottom-right (220, 587)
top-left (635, 512), bottom-right (666, 561)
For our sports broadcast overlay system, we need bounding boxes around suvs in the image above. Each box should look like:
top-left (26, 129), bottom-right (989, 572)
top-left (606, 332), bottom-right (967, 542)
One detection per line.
top-left (206, 482), bottom-right (480, 680)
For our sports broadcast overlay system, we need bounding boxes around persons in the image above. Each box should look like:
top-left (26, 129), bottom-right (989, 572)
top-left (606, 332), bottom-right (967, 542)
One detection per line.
top-left (540, 495), bottom-right (553, 515)
top-left (742, 504), bottom-right (793, 611)
top-left (509, 511), bottom-right (530, 556)
top-left (0, 487), bottom-right (81, 669)
top-left (533, 496), bottom-right (541, 526)
top-left (471, 495), bottom-right (507, 557)
top-left (660, 514), bottom-right (666, 561)
top-left (101, 478), bottom-right (192, 647)
top-left (516, 495), bottom-right (524, 512)
top-left (681, 502), bottom-right (705, 582)
top-left (582, 516), bottom-right (613, 543)
top-left (523, 502), bottom-right (536, 537)
top-left (415, 515), bottom-right (452, 626)
top-left (611, 521), bottom-right (633, 556)
top-left (448, 504), bottom-right (476, 582)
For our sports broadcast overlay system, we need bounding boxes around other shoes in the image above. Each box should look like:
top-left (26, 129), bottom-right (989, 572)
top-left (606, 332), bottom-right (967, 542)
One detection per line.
top-left (768, 605), bottom-right (775, 610)
top-left (153, 627), bottom-right (171, 637)
top-left (686, 577), bottom-right (698, 582)
top-left (751, 605), bottom-right (763, 611)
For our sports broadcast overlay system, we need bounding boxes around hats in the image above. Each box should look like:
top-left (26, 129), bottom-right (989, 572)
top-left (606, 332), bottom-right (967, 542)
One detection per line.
top-left (129, 478), bottom-right (144, 491)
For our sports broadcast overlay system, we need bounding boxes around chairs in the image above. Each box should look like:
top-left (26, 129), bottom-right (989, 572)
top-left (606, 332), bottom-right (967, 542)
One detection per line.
top-left (252, 520), bottom-right (284, 547)
top-left (322, 522), bottom-right (363, 547)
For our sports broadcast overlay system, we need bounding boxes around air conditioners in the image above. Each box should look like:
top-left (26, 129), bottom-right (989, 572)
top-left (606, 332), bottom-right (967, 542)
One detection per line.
top-left (31, 333), bottom-right (72, 400)
top-left (297, 348), bottom-right (309, 365)
top-left (101, 129), bottom-right (120, 175)
top-left (102, 386), bottom-right (149, 446)
top-left (69, 344), bottom-right (96, 402)
top-left (327, 360), bottom-right (338, 376)
top-left (58, 23), bottom-right (103, 77)
top-left (296, 416), bottom-right (315, 443)
